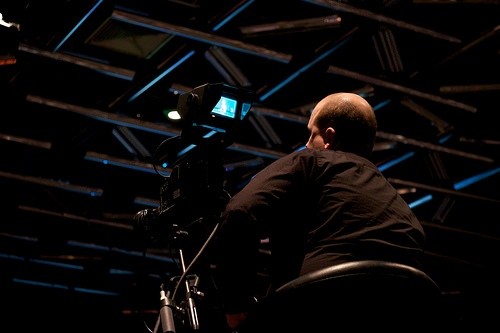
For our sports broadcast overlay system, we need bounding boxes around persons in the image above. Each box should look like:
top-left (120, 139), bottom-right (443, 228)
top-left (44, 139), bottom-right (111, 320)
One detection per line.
top-left (218, 92), bottom-right (426, 286)
